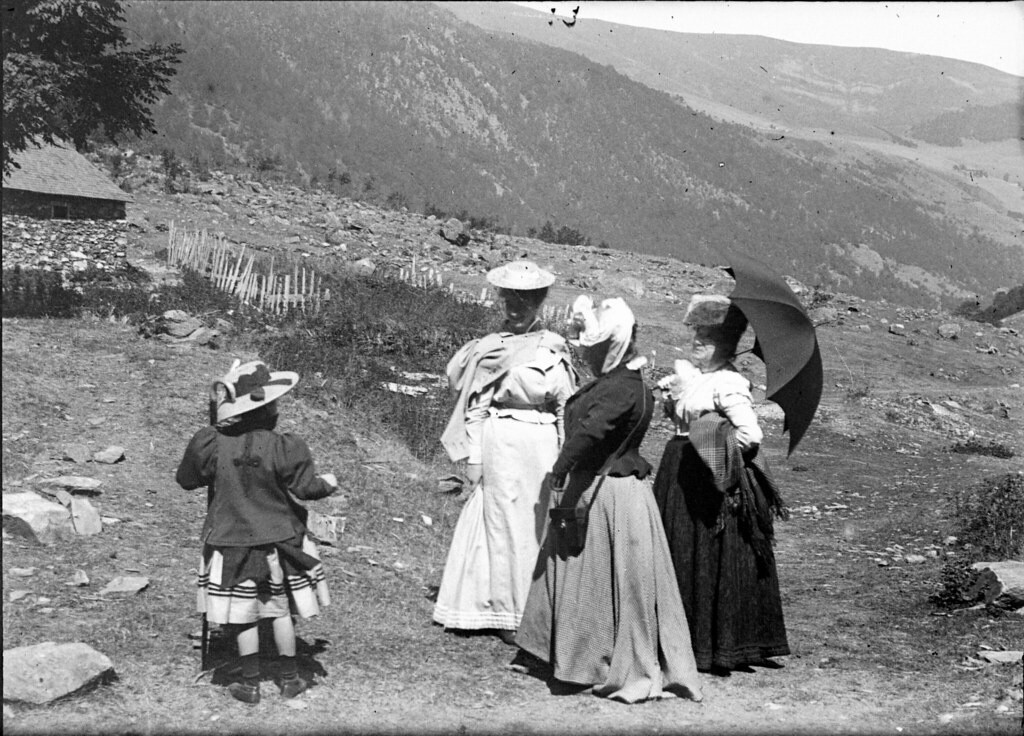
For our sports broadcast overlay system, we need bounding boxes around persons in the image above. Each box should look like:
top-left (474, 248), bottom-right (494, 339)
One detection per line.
top-left (510, 295), bottom-right (704, 704)
top-left (651, 293), bottom-right (791, 671)
top-left (432, 259), bottom-right (581, 643)
top-left (173, 359), bottom-right (338, 704)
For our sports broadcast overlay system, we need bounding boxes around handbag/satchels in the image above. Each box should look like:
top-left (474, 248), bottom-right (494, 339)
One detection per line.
top-left (550, 506), bottom-right (587, 551)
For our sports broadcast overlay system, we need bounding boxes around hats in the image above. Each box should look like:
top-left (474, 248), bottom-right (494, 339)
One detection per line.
top-left (487, 260), bottom-right (555, 289)
top-left (217, 359), bottom-right (299, 424)
top-left (683, 293), bottom-right (749, 331)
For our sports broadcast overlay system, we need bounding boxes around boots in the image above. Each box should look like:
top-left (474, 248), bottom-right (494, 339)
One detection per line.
top-left (230, 653), bottom-right (261, 703)
top-left (279, 654), bottom-right (308, 697)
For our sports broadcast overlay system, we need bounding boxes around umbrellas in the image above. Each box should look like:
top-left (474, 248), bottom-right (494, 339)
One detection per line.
top-left (718, 248), bottom-right (824, 460)
